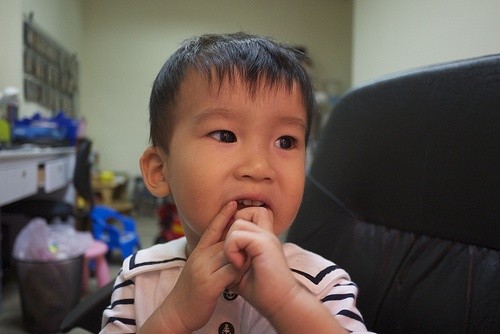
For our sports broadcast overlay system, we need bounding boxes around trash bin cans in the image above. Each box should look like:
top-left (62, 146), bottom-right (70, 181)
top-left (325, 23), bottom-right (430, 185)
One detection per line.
top-left (13, 218), bottom-right (85, 334)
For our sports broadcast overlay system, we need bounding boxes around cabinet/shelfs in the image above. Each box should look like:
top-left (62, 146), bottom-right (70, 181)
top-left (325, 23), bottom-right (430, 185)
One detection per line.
top-left (0, 148), bottom-right (77, 230)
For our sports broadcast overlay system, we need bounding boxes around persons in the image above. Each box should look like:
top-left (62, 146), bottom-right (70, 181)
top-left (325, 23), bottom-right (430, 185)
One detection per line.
top-left (99, 29), bottom-right (376, 334)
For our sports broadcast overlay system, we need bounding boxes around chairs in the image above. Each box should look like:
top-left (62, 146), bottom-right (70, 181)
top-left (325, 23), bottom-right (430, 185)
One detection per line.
top-left (89, 205), bottom-right (142, 266)
top-left (59, 55), bottom-right (500, 334)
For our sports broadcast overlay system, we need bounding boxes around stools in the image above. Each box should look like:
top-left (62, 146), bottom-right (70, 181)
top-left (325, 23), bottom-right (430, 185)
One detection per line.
top-left (81, 240), bottom-right (112, 289)
